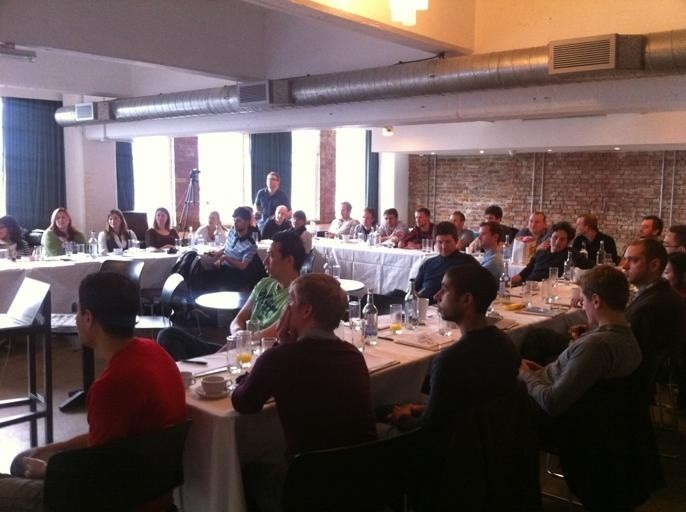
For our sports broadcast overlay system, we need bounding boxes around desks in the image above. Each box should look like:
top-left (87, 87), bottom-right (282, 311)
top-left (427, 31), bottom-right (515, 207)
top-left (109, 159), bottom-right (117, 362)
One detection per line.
top-left (0, 237), bottom-right (640, 512)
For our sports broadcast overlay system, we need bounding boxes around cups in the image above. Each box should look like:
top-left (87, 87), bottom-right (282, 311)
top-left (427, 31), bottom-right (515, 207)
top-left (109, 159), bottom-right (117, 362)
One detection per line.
top-left (337, 296), bottom-right (366, 354)
top-left (419, 298), bottom-right (429, 323)
top-left (252, 232), bottom-right (258, 246)
top-left (227, 319), bottom-right (278, 374)
top-left (522, 268), bottom-right (559, 313)
top-left (390, 304), bottom-right (402, 330)
top-left (32, 230), bottom-right (97, 262)
top-left (438, 312), bottom-right (452, 338)
top-left (181, 372), bottom-right (196, 388)
top-left (465, 247), bottom-right (473, 255)
top-left (200, 376), bottom-right (233, 393)
top-left (324, 232), bottom-right (364, 243)
top-left (113, 239), bottom-right (140, 255)
top-left (422, 239), bottom-right (433, 254)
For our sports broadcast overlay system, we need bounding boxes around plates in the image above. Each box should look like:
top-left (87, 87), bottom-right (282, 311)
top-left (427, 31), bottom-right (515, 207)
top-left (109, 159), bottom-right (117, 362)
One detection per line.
top-left (195, 384), bottom-right (230, 398)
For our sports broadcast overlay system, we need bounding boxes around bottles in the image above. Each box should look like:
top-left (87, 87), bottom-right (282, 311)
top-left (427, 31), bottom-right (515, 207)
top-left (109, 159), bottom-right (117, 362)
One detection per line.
top-left (368, 224), bottom-right (381, 247)
top-left (310, 221), bottom-right (317, 240)
top-left (175, 224), bottom-right (223, 253)
top-left (500, 235), bottom-right (512, 301)
top-left (564, 241), bottom-right (616, 282)
top-left (404, 279), bottom-right (420, 330)
top-left (363, 288), bottom-right (379, 346)
top-left (0, 242), bottom-right (9, 264)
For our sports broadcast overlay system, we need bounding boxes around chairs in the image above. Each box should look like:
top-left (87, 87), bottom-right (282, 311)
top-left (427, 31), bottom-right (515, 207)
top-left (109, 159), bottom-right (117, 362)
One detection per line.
top-left (646, 346), bottom-right (682, 459)
top-left (26, 230), bottom-right (43, 245)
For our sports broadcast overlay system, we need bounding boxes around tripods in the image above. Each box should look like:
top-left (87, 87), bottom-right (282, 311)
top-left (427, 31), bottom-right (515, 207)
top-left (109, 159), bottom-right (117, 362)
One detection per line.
top-left (176, 178), bottom-right (211, 240)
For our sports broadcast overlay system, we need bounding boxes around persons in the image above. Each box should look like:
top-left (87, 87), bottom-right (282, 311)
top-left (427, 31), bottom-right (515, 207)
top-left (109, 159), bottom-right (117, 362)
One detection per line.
top-left (325, 202), bottom-right (410, 242)
top-left (373, 264), bottom-right (523, 442)
top-left (0, 271), bottom-right (186, 512)
top-left (507, 211), bottom-right (685, 414)
top-left (399, 205), bottom-right (518, 306)
top-left (1, 207), bottom-right (179, 259)
top-left (252, 169), bottom-right (293, 228)
top-left (221, 231), bottom-right (377, 450)
top-left (193, 205), bottom-right (312, 269)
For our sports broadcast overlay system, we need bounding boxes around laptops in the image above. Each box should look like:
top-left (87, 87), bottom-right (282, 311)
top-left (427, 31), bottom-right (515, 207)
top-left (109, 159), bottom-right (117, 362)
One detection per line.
top-left (0, 277), bottom-right (51, 330)
